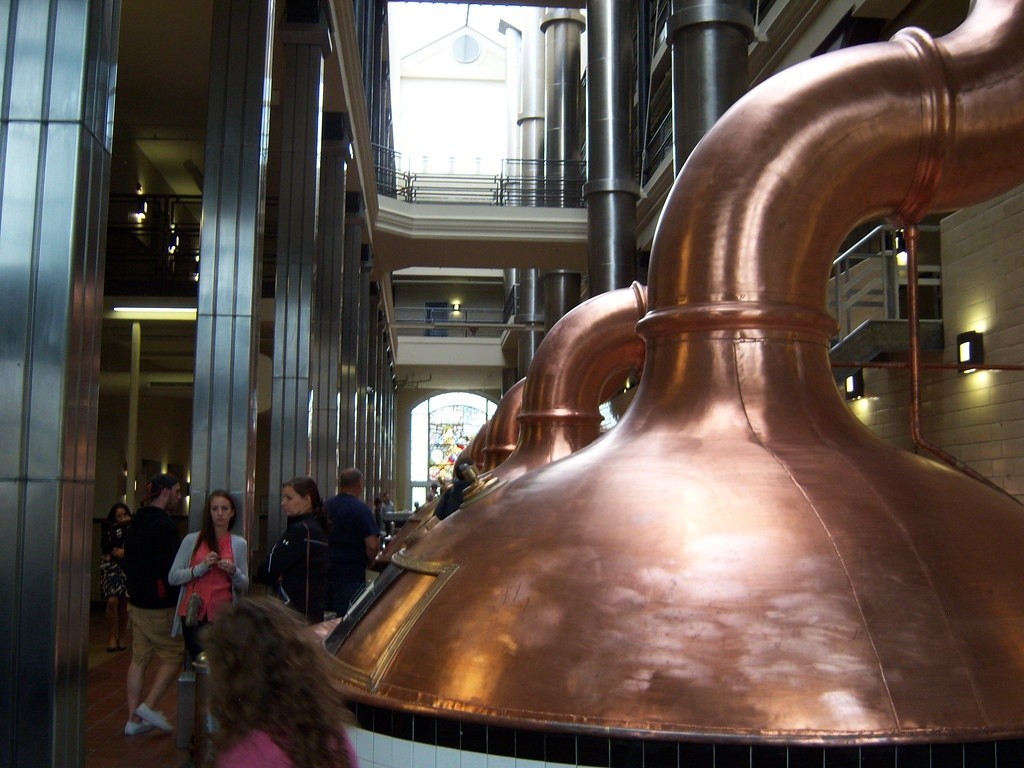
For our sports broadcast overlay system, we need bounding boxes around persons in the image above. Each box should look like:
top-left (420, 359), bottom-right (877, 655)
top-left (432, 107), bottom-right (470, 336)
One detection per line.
top-left (324, 467), bottom-right (379, 616)
top-left (426, 484), bottom-right (438, 502)
top-left (382, 493), bottom-right (394, 537)
top-left (166, 488), bottom-right (249, 674)
top-left (101, 502), bottom-right (131, 651)
top-left (267, 476), bottom-right (328, 624)
top-left (119, 473), bottom-right (183, 737)
top-left (435, 457), bottom-right (473, 520)
top-left (192, 597), bottom-right (359, 768)
top-left (371, 498), bottom-right (386, 531)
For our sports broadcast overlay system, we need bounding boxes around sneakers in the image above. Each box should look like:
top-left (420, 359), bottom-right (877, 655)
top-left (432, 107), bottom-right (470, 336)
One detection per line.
top-left (134, 702), bottom-right (172, 733)
top-left (124, 720), bottom-right (153, 736)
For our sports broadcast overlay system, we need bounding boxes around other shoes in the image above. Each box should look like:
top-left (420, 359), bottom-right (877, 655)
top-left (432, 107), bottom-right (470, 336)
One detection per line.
top-left (118, 646), bottom-right (126, 651)
top-left (107, 647), bottom-right (117, 652)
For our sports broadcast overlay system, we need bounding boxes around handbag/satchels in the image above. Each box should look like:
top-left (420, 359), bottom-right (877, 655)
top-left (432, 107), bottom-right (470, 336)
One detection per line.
top-left (185, 567), bottom-right (202, 627)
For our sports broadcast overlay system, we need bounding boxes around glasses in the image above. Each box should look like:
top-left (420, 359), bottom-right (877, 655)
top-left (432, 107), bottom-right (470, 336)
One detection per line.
top-left (192, 651), bottom-right (210, 674)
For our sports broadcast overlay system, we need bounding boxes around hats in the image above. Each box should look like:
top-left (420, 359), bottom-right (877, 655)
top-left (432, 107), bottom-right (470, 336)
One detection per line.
top-left (144, 475), bottom-right (177, 500)
